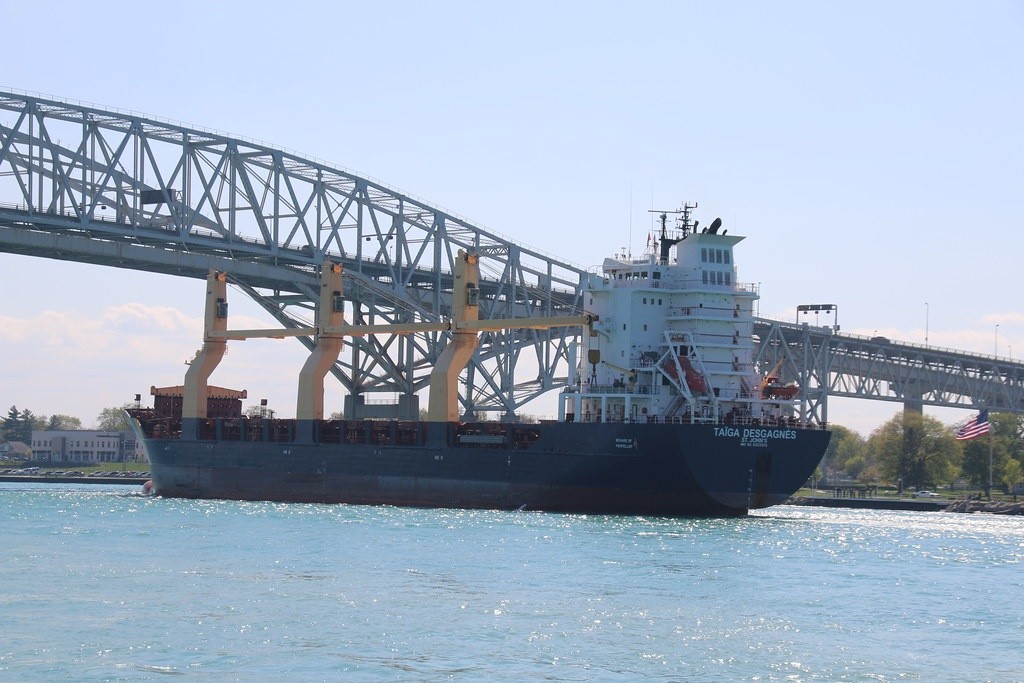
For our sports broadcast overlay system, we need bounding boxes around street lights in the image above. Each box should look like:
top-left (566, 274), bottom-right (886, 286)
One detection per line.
top-left (925, 302), bottom-right (928, 350)
top-left (994, 324), bottom-right (999, 359)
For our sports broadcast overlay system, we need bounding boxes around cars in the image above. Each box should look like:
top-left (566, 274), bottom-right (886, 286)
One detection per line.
top-left (913, 490), bottom-right (940, 498)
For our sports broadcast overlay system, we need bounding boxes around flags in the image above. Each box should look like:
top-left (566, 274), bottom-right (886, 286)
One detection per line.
top-left (955, 408), bottom-right (990, 441)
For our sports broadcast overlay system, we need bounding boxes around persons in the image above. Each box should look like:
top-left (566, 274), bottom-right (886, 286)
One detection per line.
top-left (577, 379), bottom-right (582, 394)
top-left (833, 486), bottom-right (878, 499)
top-left (1013, 494), bottom-right (1016, 502)
top-left (639, 352), bottom-right (645, 368)
top-left (967, 492), bottom-right (982, 501)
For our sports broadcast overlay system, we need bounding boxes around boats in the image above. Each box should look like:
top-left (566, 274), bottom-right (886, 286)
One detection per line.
top-left (125, 205), bottom-right (842, 516)
